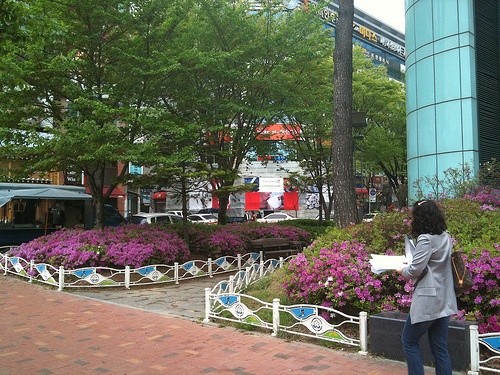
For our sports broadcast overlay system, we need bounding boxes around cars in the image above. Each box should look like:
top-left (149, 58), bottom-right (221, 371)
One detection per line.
top-left (132, 213), bottom-right (174, 227)
top-left (186, 214), bottom-right (218, 224)
top-left (256, 213), bottom-right (296, 224)
top-left (314, 212), bottom-right (380, 224)
top-left (169, 211), bottom-right (186, 217)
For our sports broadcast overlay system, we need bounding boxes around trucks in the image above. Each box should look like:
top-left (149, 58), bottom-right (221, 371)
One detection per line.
top-left (0, 184), bottom-right (129, 247)
top-left (197, 208), bottom-right (247, 225)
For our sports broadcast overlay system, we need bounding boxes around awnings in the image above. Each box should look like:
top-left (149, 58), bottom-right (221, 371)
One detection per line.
top-left (1, 182), bottom-right (93, 209)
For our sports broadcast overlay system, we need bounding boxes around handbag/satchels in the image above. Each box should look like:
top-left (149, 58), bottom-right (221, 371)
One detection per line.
top-left (450, 245), bottom-right (475, 295)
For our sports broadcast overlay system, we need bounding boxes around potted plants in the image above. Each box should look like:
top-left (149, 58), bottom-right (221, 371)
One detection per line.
top-left (32, 220), bottom-right (43, 229)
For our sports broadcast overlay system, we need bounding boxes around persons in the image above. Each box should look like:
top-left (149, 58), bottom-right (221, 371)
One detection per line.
top-left (55, 210), bottom-right (66, 227)
top-left (256, 211), bottom-right (262, 219)
top-left (244, 211), bottom-right (249, 221)
top-left (394, 199), bottom-right (458, 375)
top-left (250, 211), bottom-right (255, 221)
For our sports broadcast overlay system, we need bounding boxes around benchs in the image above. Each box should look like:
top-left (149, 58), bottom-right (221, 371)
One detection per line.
top-left (250, 237), bottom-right (302, 260)
top-left (369, 312), bottom-right (479, 370)
